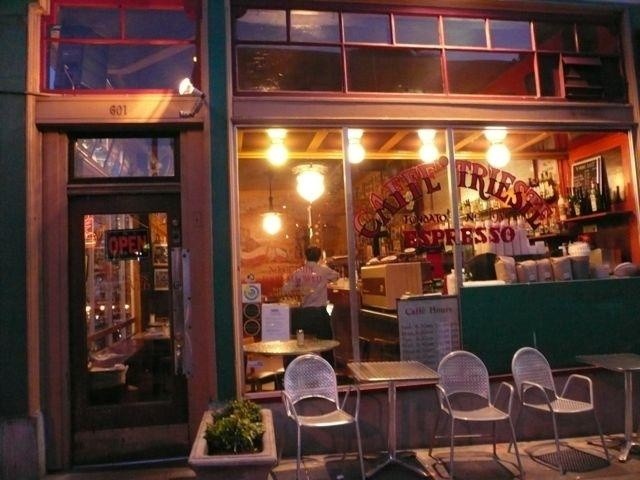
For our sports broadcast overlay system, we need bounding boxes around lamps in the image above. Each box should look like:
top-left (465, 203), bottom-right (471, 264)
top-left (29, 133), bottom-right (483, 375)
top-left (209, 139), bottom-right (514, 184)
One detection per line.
top-left (260, 125), bottom-right (512, 236)
top-left (176, 78), bottom-right (208, 117)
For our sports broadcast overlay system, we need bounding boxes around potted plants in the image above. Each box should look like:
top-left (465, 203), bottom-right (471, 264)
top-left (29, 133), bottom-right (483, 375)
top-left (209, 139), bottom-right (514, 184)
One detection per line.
top-left (188, 398), bottom-right (277, 480)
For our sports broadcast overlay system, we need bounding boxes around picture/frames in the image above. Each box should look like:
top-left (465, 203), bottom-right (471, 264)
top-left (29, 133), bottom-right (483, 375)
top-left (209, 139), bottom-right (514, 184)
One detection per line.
top-left (571, 155), bottom-right (602, 197)
top-left (153, 244), bottom-right (169, 266)
top-left (154, 270), bottom-right (171, 291)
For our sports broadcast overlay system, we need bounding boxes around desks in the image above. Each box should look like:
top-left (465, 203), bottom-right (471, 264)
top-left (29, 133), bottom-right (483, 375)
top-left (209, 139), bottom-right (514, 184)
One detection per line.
top-left (576, 353), bottom-right (640, 462)
top-left (131, 317), bottom-right (172, 395)
top-left (347, 360), bottom-right (442, 477)
top-left (243, 339), bottom-right (340, 389)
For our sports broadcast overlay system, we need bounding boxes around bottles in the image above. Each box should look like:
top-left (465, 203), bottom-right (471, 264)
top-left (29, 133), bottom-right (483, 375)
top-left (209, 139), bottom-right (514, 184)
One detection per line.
top-left (296, 329), bottom-right (305, 346)
top-left (558, 181), bottom-right (624, 218)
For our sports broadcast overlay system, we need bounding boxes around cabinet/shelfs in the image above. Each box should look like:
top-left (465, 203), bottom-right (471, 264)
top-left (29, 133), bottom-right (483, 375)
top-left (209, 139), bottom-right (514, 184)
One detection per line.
top-left (450, 179), bottom-right (562, 253)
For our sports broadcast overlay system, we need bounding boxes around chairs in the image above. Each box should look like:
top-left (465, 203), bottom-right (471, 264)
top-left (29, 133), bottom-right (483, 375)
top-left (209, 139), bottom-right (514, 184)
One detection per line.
top-left (507, 347), bottom-right (611, 476)
top-left (239, 338), bottom-right (279, 392)
top-left (428, 350), bottom-right (525, 480)
top-left (280, 354), bottom-right (368, 480)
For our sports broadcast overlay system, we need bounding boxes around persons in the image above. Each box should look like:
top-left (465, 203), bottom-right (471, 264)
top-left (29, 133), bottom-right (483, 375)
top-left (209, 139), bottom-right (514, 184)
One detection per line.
top-left (284, 245), bottom-right (339, 368)
